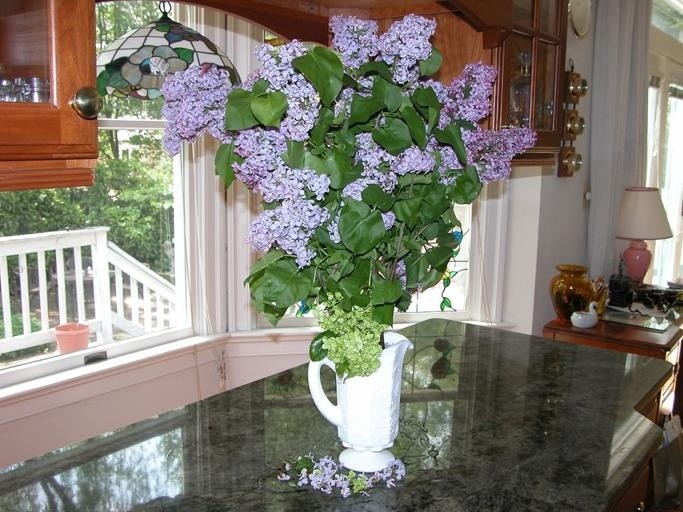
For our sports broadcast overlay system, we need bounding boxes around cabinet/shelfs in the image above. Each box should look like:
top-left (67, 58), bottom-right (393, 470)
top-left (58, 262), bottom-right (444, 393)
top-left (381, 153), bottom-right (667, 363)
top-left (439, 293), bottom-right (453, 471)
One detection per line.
top-left (328, 0), bottom-right (569, 166)
top-left (0, 0), bottom-right (104, 191)
top-left (542, 310), bottom-right (683, 430)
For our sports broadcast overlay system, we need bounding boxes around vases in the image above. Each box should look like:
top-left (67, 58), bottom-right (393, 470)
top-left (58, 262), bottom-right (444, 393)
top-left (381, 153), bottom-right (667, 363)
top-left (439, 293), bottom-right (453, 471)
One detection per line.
top-left (53, 324), bottom-right (91, 355)
top-left (307, 331), bottom-right (416, 475)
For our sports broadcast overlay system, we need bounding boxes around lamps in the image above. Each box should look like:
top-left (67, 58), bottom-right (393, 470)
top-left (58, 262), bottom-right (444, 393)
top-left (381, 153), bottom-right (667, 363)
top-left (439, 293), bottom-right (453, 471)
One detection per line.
top-left (613, 186), bottom-right (674, 284)
top-left (95, 1), bottom-right (242, 102)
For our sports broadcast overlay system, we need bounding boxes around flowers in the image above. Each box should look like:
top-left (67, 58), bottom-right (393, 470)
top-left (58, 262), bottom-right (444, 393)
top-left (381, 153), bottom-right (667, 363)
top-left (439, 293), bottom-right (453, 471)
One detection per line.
top-left (158, 13), bottom-right (541, 384)
